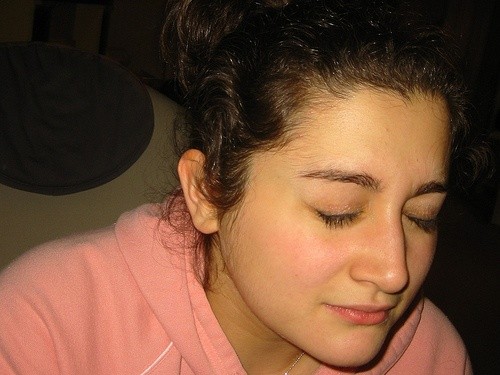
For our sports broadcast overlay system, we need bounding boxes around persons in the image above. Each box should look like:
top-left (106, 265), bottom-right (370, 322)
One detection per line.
top-left (1, 2), bottom-right (486, 375)
